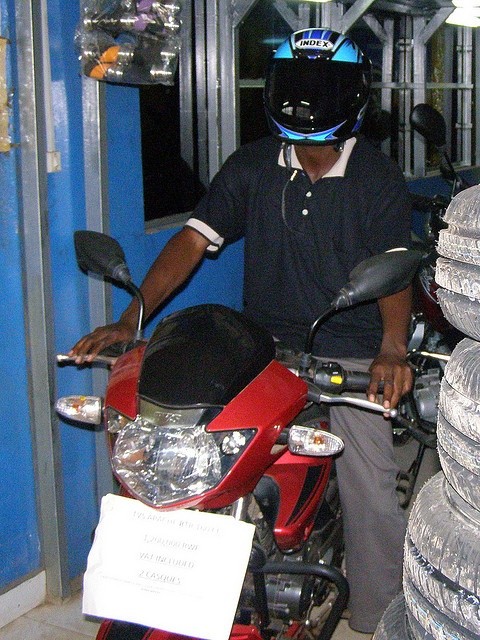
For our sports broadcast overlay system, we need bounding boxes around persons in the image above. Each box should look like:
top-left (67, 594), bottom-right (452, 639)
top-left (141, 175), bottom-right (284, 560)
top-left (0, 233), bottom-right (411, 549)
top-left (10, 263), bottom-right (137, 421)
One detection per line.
top-left (67, 27), bottom-right (415, 635)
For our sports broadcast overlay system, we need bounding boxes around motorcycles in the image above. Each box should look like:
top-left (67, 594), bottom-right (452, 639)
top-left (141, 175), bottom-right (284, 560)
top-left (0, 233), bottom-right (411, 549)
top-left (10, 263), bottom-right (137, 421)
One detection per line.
top-left (54, 228), bottom-right (434, 640)
top-left (394, 102), bottom-right (469, 508)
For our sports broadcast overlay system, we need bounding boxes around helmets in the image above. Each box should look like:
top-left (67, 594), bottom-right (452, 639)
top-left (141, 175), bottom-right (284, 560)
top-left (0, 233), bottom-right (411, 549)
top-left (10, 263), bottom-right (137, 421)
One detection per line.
top-left (262, 27), bottom-right (374, 147)
